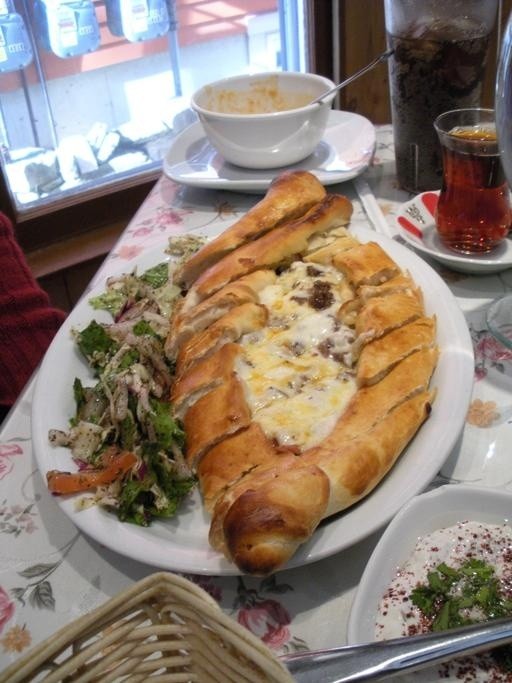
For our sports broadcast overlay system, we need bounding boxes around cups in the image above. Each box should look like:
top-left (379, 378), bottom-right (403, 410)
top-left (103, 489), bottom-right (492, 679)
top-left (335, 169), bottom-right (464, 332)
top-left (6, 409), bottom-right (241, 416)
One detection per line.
top-left (432, 106), bottom-right (512, 257)
top-left (384, 0), bottom-right (502, 195)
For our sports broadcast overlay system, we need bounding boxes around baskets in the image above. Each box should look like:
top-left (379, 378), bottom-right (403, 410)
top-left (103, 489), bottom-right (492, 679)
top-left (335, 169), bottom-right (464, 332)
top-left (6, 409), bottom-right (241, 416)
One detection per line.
top-left (0, 570), bottom-right (298, 681)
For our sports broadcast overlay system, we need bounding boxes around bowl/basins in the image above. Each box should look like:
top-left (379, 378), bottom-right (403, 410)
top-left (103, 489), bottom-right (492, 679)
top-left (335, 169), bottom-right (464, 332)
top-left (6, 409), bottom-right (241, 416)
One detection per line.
top-left (187, 67), bottom-right (338, 170)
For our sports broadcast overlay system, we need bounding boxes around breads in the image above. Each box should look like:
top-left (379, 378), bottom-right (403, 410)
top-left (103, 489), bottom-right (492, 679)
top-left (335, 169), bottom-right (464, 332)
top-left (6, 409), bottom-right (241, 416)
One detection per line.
top-left (164, 173), bottom-right (439, 579)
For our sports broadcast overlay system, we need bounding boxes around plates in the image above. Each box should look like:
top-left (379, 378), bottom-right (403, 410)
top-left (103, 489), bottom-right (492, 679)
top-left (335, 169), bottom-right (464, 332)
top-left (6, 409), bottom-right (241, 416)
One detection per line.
top-left (27, 214), bottom-right (475, 580)
top-left (395, 186), bottom-right (512, 276)
top-left (345, 483), bottom-right (510, 681)
top-left (162, 106), bottom-right (378, 194)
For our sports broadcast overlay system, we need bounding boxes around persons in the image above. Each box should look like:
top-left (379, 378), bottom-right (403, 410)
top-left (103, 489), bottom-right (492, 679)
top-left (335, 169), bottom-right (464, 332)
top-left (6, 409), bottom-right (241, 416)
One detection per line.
top-left (0, 216), bottom-right (66, 409)
